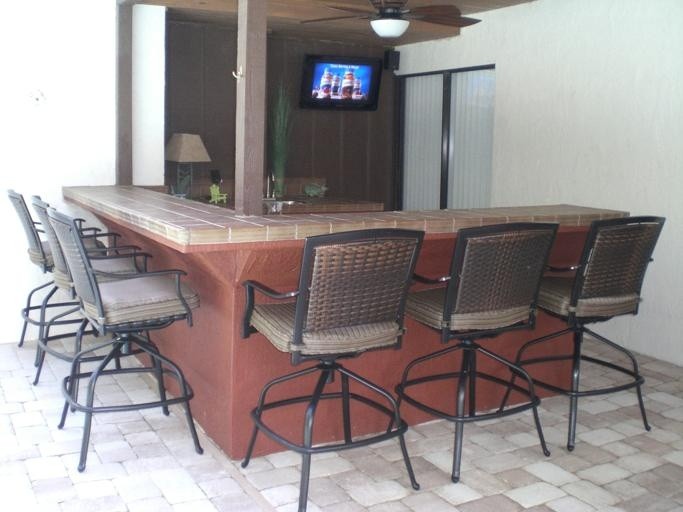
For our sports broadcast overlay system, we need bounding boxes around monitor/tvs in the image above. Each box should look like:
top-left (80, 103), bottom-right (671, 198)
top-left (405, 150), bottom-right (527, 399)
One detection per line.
top-left (299, 54), bottom-right (382, 111)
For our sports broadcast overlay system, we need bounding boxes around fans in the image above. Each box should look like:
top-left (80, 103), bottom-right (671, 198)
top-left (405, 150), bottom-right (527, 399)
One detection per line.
top-left (297, 0), bottom-right (482, 28)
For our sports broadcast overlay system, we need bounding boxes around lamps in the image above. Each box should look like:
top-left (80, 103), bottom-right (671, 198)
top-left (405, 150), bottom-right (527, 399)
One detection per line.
top-left (163, 132), bottom-right (211, 196)
top-left (369, 19), bottom-right (410, 38)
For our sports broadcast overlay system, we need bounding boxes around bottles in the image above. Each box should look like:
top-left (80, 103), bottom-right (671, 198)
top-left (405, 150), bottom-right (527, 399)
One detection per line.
top-left (318, 67), bottom-right (362, 100)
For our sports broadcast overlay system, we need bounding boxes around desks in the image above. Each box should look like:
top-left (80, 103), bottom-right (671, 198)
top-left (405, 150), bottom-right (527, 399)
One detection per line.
top-left (188, 194), bottom-right (384, 213)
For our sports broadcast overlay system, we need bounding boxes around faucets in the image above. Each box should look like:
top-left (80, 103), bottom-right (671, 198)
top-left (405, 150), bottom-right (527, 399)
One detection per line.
top-left (266, 166), bottom-right (275, 198)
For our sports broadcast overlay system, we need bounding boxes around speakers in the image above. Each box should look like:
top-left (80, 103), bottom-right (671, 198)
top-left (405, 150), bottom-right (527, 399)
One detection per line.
top-left (384, 50), bottom-right (400, 71)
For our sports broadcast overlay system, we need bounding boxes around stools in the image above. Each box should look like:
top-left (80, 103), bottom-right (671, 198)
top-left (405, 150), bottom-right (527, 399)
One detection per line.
top-left (7, 190), bottom-right (203, 473)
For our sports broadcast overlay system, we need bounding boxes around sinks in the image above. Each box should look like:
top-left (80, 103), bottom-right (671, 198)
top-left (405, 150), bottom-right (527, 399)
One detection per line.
top-left (261, 200), bottom-right (312, 206)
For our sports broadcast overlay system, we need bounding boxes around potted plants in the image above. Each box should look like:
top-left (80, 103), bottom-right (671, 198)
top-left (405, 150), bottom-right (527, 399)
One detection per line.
top-left (268, 77), bottom-right (296, 202)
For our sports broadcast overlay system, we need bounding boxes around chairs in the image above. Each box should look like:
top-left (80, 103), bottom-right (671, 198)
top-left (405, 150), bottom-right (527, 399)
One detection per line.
top-left (387, 219), bottom-right (560, 483)
top-left (238, 228), bottom-right (419, 512)
top-left (494, 215), bottom-right (670, 452)
